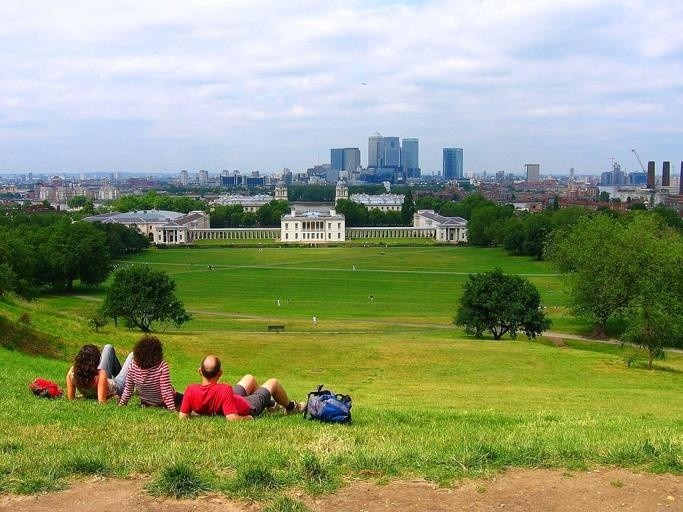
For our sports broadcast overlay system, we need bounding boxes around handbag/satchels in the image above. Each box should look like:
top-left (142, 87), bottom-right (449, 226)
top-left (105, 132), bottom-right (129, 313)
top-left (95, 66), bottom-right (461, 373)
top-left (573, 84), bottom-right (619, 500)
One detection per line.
top-left (304, 385), bottom-right (352, 424)
top-left (29, 378), bottom-right (64, 399)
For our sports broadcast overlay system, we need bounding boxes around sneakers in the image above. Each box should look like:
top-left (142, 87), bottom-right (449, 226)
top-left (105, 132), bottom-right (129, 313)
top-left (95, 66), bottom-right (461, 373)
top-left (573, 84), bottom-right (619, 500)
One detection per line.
top-left (284, 400), bottom-right (306, 416)
top-left (267, 399), bottom-right (284, 413)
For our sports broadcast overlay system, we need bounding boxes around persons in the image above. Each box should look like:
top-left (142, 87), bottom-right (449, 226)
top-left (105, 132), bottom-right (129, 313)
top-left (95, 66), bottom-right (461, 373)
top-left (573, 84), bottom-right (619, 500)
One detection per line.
top-left (66, 342), bottom-right (134, 405)
top-left (178, 354), bottom-right (306, 423)
top-left (117, 336), bottom-right (183, 416)
